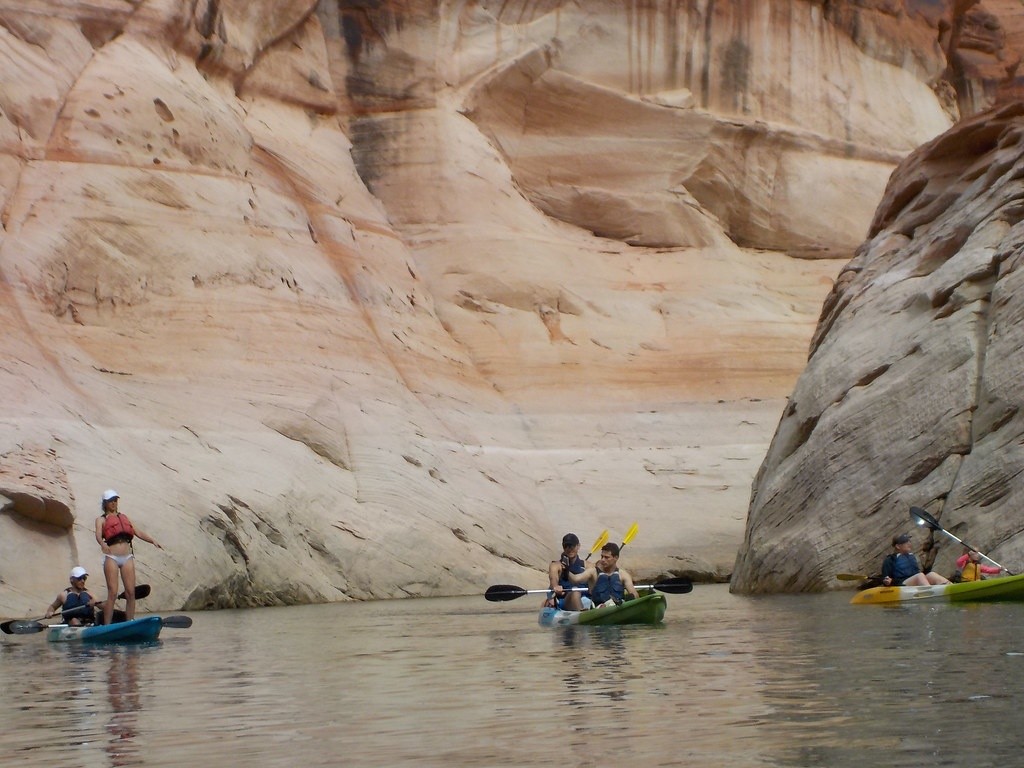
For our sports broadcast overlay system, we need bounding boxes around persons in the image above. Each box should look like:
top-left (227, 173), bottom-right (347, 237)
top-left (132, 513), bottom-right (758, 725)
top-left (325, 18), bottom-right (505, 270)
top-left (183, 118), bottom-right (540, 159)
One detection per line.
top-left (96, 489), bottom-right (164, 625)
top-left (956, 546), bottom-right (1004, 582)
top-left (46, 567), bottom-right (103, 625)
top-left (561, 543), bottom-right (639, 607)
top-left (881, 533), bottom-right (953, 586)
top-left (549, 532), bottom-right (606, 611)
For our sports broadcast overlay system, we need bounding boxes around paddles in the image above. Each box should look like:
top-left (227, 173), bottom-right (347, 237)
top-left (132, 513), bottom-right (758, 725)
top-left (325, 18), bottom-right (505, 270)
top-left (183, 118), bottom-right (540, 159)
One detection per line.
top-left (9, 614), bottom-right (193, 635)
top-left (910, 506), bottom-right (1015, 576)
top-left (618, 519), bottom-right (640, 552)
top-left (483, 575), bottom-right (694, 603)
top-left (0, 583), bottom-right (151, 635)
top-left (584, 528), bottom-right (609, 561)
top-left (837, 572), bottom-right (901, 582)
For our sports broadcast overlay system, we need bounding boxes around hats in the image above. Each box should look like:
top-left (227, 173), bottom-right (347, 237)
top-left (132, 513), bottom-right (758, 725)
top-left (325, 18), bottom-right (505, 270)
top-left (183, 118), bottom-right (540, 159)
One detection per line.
top-left (70, 567), bottom-right (89, 578)
top-left (102, 490), bottom-right (120, 500)
top-left (892, 535), bottom-right (912, 546)
top-left (563, 533), bottom-right (579, 545)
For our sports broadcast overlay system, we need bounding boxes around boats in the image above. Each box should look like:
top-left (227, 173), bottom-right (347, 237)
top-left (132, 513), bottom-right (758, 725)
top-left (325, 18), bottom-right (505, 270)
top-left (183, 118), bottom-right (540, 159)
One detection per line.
top-left (538, 593), bottom-right (667, 628)
top-left (47, 615), bottom-right (164, 644)
top-left (623, 586), bottom-right (656, 600)
top-left (848, 573), bottom-right (1024, 607)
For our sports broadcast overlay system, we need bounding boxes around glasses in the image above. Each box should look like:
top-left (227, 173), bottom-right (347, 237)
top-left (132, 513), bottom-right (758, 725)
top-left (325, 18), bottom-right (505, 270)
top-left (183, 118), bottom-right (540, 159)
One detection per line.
top-left (563, 544), bottom-right (576, 549)
top-left (72, 577), bottom-right (87, 580)
